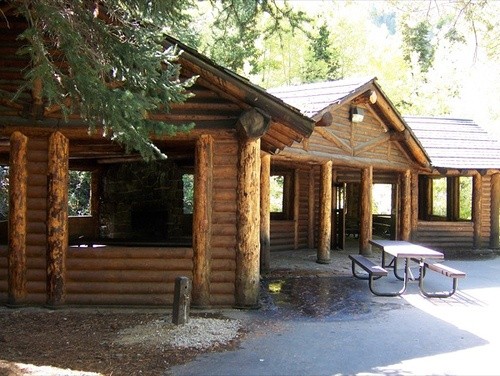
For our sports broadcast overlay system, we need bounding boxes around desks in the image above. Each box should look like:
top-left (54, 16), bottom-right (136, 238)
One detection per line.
top-left (369, 240), bottom-right (444, 297)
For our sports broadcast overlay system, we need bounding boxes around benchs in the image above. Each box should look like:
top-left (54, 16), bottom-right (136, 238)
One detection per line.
top-left (349, 254), bottom-right (388, 296)
top-left (411, 258), bottom-right (465, 297)
